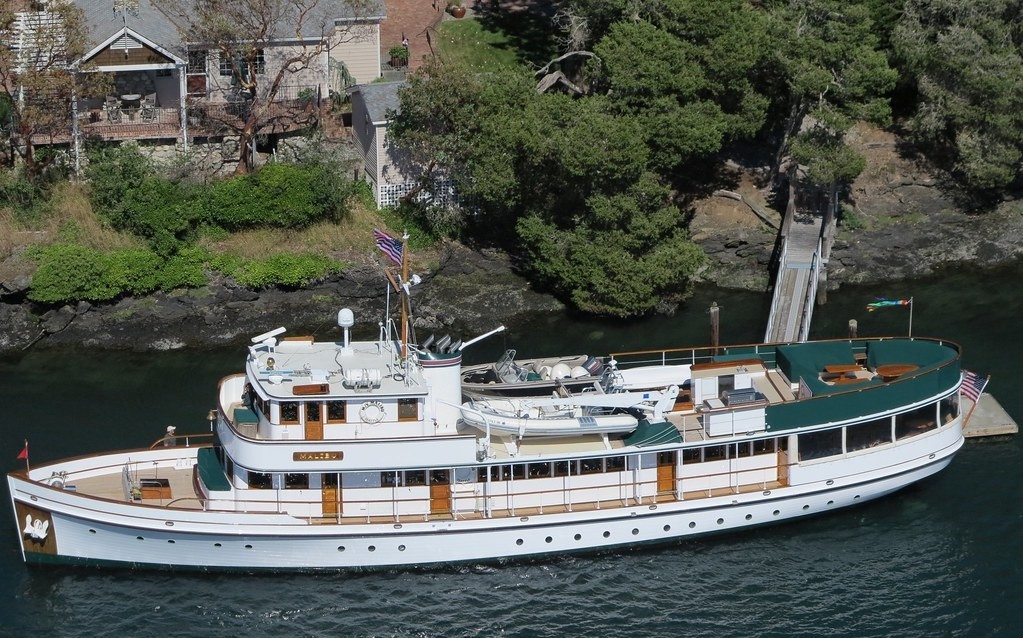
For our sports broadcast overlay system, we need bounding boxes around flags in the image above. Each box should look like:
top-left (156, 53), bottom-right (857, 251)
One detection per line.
top-left (374, 227), bottom-right (404, 266)
top-left (961, 371), bottom-right (987, 403)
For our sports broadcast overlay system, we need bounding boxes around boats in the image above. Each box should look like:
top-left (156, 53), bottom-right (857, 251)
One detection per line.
top-left (461, 348), bottom-right (608, 397)
top-left (6, 230), bottom-right (965, 572)
top-left (461, 385), bottom-right (679, 439)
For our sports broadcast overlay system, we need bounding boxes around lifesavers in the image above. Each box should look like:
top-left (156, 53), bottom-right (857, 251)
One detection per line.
top-left (48, 477), bottom-right (65, 488)
top-left (359, 400), bottom-right (385, 424)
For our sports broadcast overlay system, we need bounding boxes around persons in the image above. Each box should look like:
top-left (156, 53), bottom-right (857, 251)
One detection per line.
top-left (241, 383), bottom-right (256, 407)
top-left (163, 426), bottom-right (176, 446)
